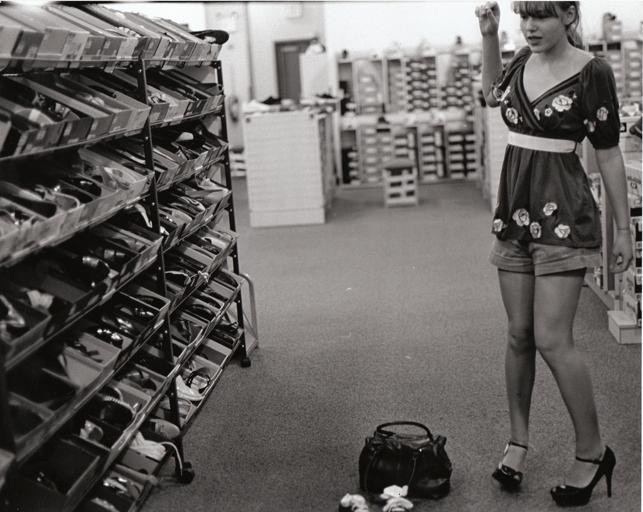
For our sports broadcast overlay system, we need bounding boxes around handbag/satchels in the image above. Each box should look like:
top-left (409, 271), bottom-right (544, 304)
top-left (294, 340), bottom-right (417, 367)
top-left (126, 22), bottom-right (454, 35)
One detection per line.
top-left (359, 420), bottom-right (452, 503)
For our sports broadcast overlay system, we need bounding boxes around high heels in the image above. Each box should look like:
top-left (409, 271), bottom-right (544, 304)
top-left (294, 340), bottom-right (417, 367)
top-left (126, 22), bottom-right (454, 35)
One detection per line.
top-left (550, 445), bottom-right (616, 507)
top-left (492, 441), bottom-right (527, 487)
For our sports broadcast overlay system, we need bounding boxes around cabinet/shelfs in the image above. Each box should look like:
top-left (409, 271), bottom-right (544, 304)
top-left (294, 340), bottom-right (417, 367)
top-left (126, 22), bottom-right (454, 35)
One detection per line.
top-left (236, 8), bottom-right (642, 347)
top-left (1, 1), bottom-right (262, 512)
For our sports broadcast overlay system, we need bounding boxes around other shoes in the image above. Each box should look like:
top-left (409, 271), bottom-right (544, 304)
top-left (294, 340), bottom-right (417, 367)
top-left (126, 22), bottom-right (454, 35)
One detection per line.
top-left (0, 71), bottom-right (240, 512)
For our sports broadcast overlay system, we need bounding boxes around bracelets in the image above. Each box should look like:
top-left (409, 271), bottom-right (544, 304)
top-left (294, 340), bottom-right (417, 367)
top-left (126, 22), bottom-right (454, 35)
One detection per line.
top-left (616, 225), bottom-right (631, 233)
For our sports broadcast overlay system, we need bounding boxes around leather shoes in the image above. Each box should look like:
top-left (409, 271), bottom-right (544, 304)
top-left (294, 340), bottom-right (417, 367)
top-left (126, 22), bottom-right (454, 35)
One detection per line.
top-left (192, 31), bottom-right (229, 46)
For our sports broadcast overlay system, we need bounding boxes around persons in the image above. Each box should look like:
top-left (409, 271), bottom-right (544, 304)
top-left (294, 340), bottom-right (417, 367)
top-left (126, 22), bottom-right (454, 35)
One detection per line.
top-left (471, 1), bottom-right (635, 508)
